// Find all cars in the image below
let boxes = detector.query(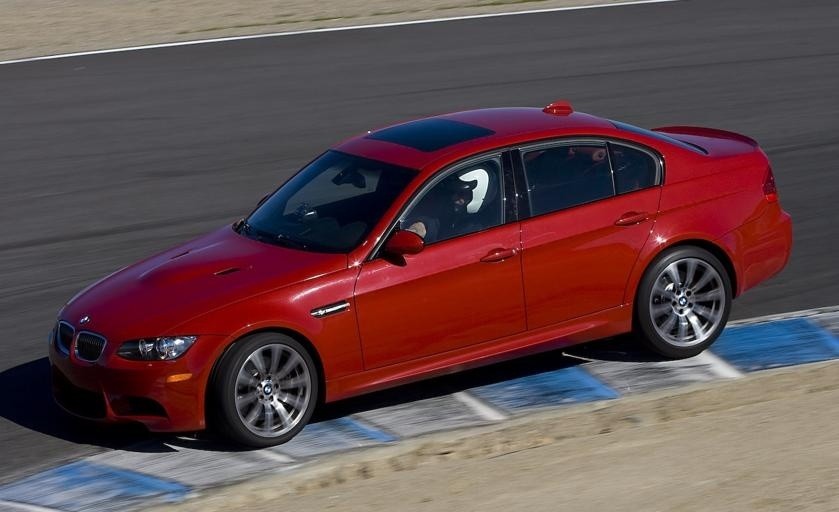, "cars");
[48,99,794,449]
